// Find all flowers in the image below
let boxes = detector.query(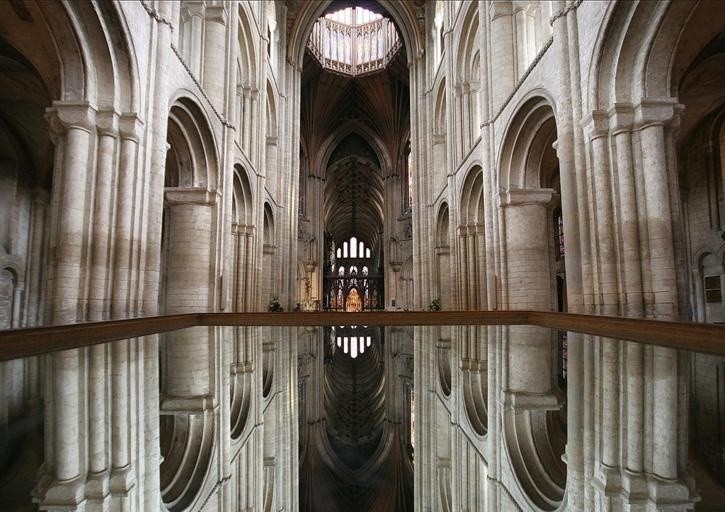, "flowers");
[428,297,441,311]
[267,296,284,311]
